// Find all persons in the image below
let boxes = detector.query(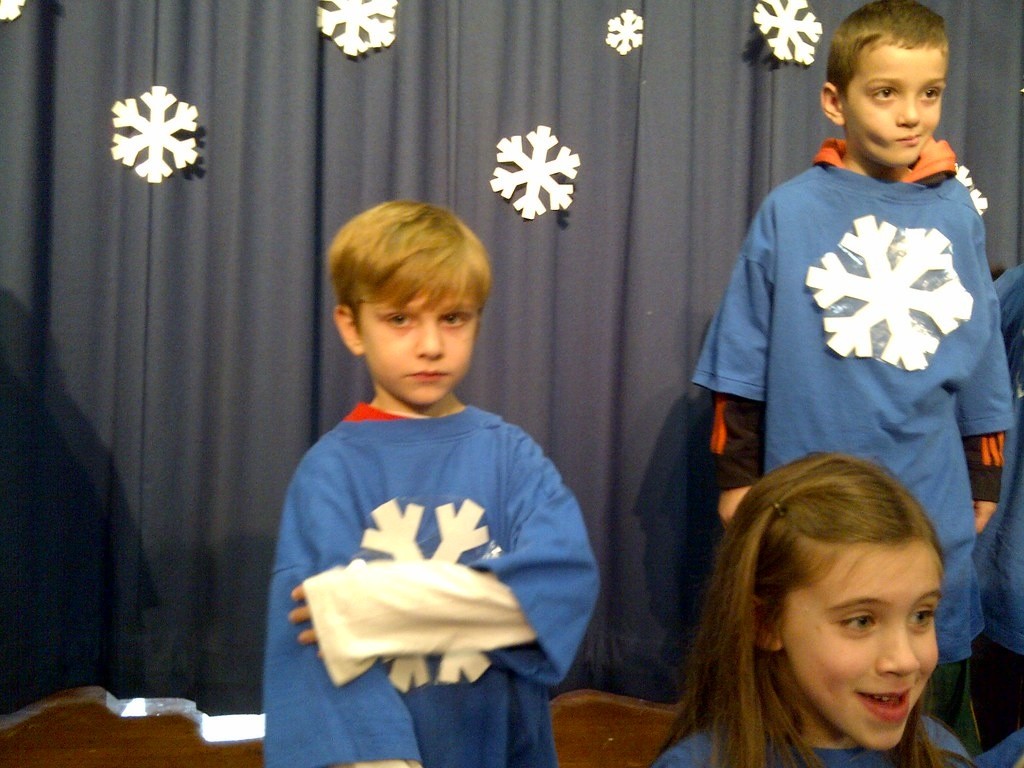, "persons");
[690,1,1016,757]
[973,259,1024,753]
[654,452,975,768]
[264,201,600,767]
